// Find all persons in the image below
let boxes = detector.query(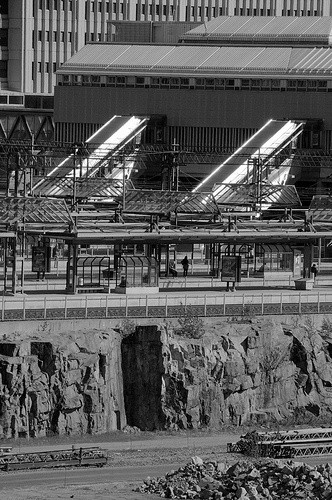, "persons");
[118,276,127,288]
[182,255,190,276]
[169,261,178,278]
[311,263,318,278]
[258,262,267,272]
